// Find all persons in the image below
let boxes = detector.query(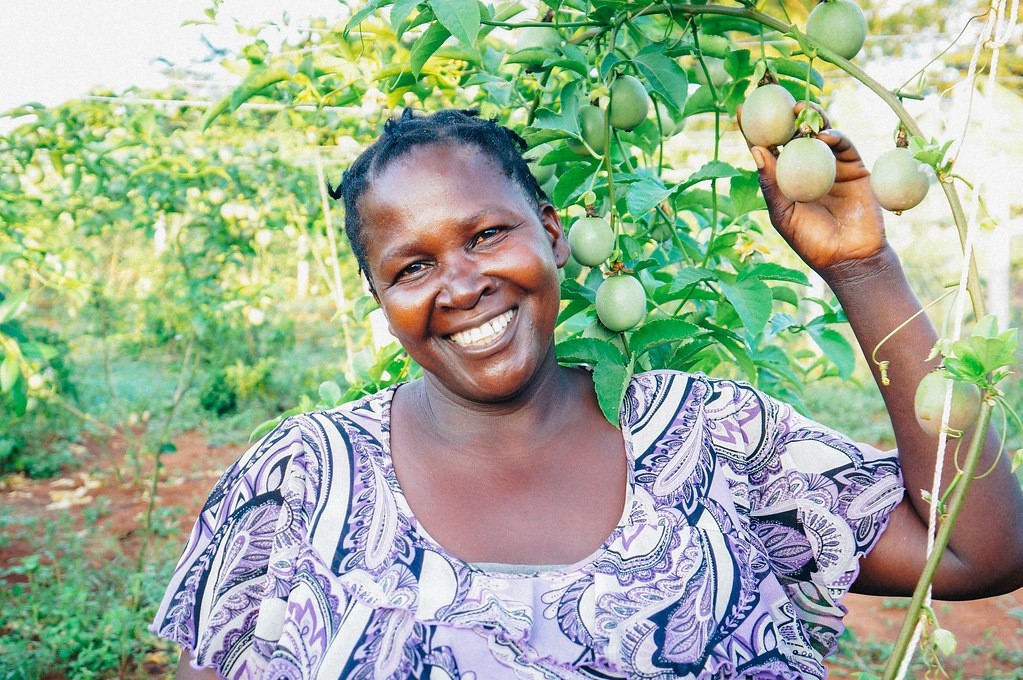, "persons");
[148,102,1023,680]
